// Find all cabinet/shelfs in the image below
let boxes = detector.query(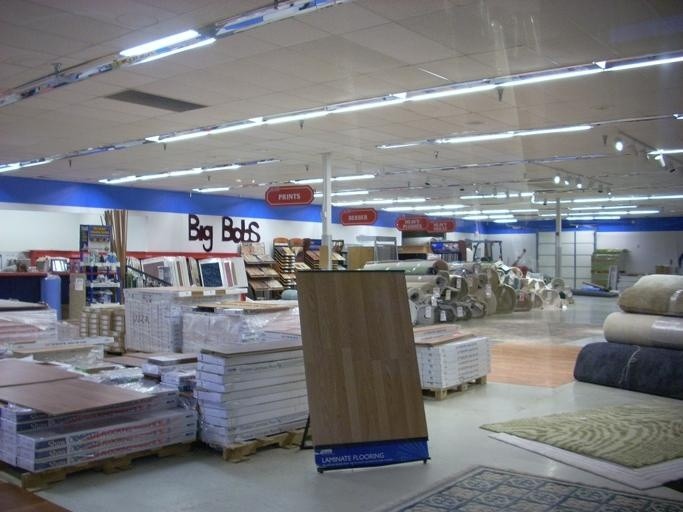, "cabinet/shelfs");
[240,238,344,300]
